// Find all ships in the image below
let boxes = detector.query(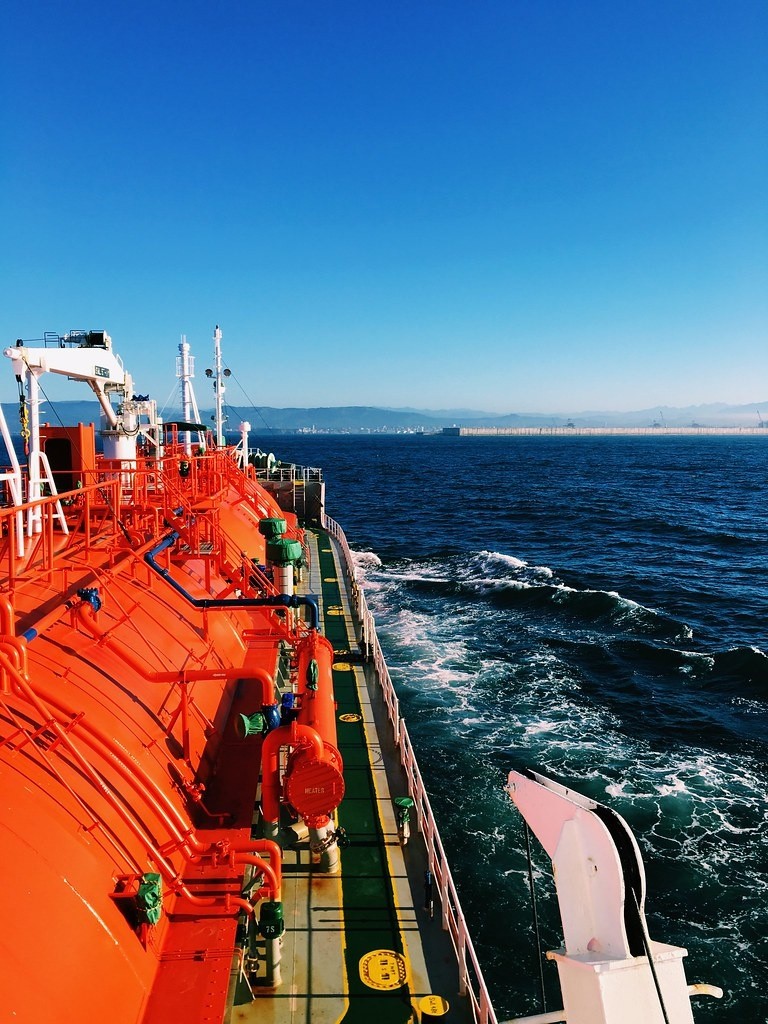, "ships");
[0,322,725,1024]
